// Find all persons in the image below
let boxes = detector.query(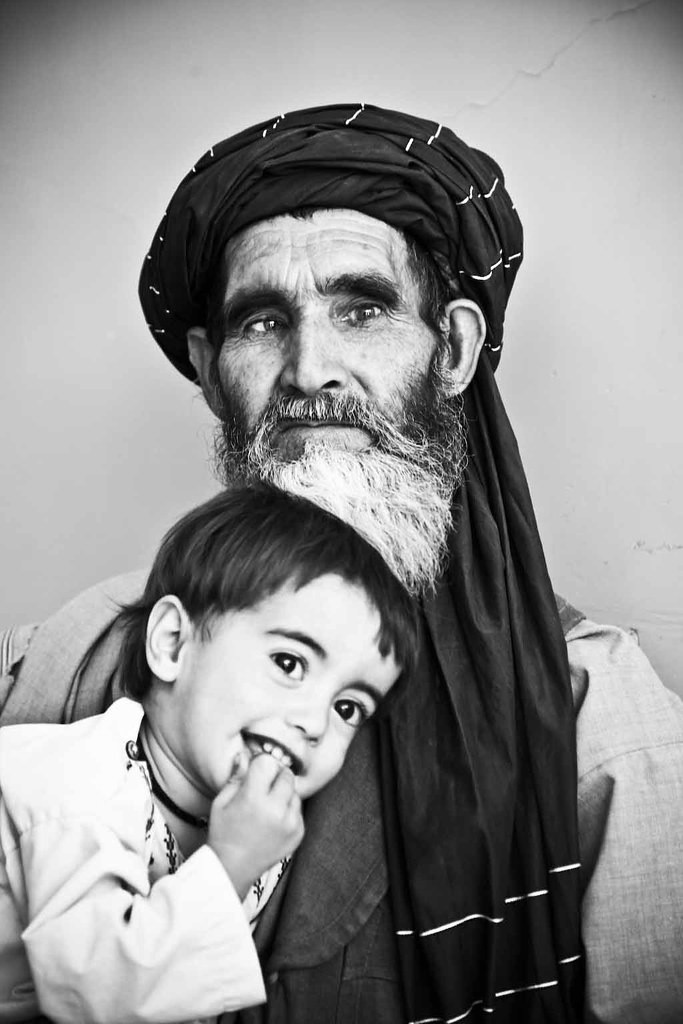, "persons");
[0,100,683,1024]
[0,480,424,1024]
